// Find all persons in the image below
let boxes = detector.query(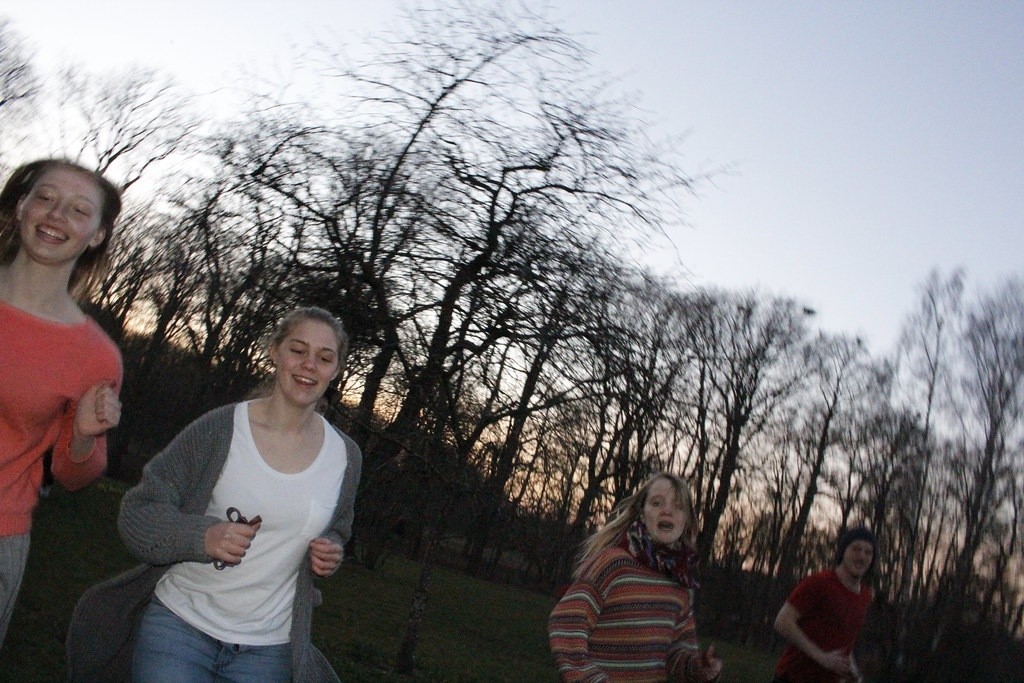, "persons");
[65,308,363,682]
[549,473,724,683]
[772,529,877,683]
[0,158,123,645]
